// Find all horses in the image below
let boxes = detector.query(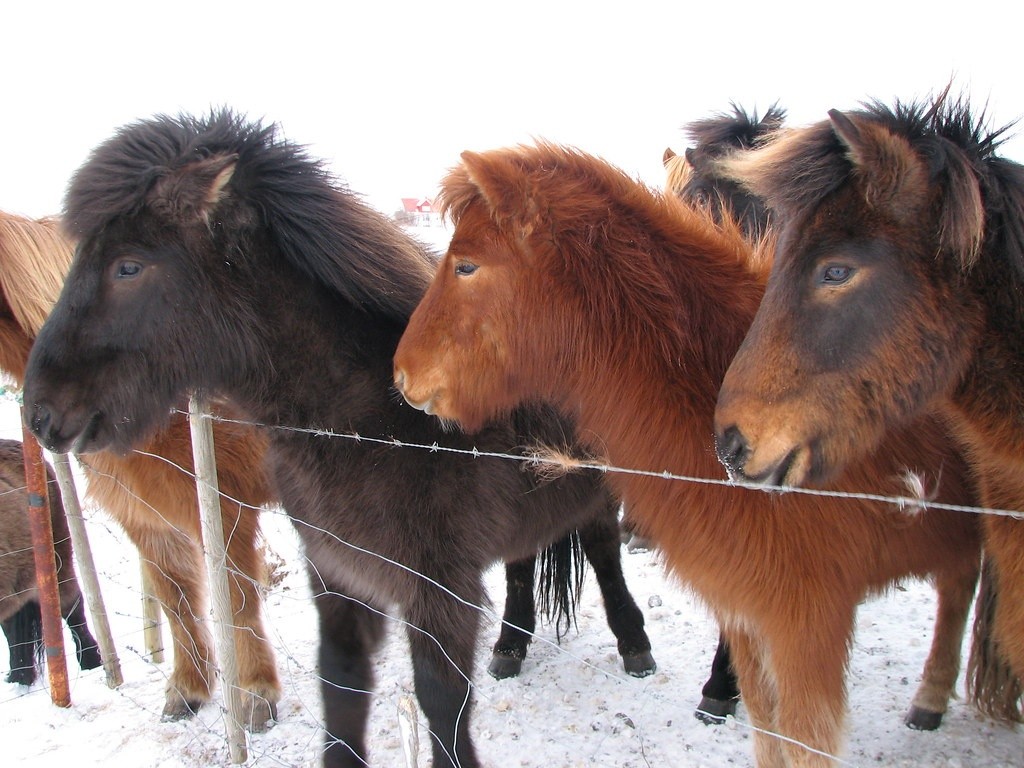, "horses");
[662,74,1021,724]
[0,207,281,733]
[21,108,656,767]
[0,438,105,688]
[388,130,1024,768]
